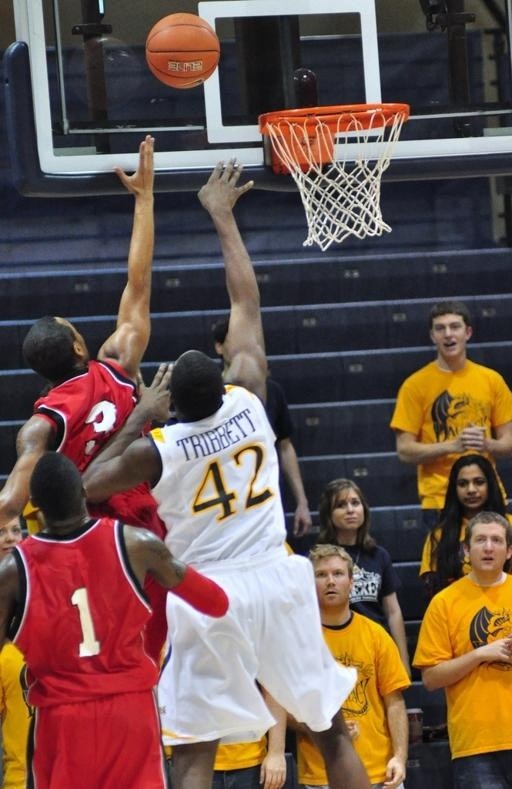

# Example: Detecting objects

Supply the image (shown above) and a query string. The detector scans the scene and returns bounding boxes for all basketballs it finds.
[146,13,220,89]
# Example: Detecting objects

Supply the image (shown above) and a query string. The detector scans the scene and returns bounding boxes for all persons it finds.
[164,478,413,788]
[411,510,512,789]
[1,133,168,660]
[1,452,229,788]
[416,453,512,598]
[84,156,373,788]
[389,300,512,529]
[0,500,44,788]
[211,316,312,537]
